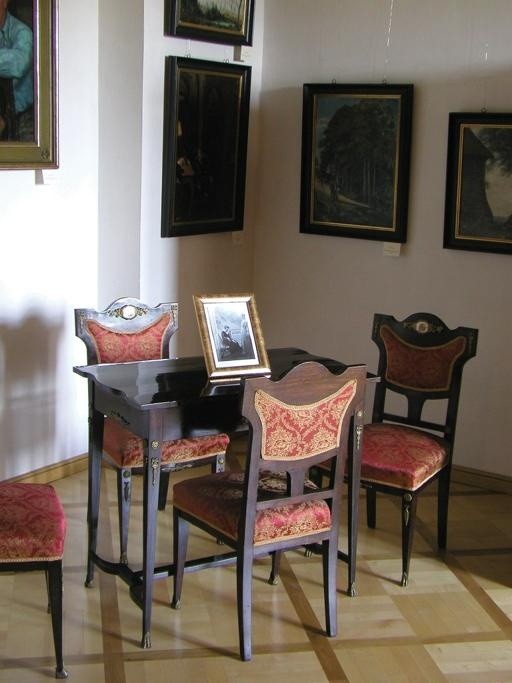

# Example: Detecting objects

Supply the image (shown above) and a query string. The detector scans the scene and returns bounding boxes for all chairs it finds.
[74,295,229,566]
[170,360,367,661]
[304,313,480,586]
[1,484,68,678]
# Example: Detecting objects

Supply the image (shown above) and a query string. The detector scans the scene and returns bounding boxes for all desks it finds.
[73,345,381,649]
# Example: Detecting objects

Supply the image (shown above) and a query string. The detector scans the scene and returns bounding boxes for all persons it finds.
[240,312,255,356]
[0,0,35,142]
[220,324,243,356]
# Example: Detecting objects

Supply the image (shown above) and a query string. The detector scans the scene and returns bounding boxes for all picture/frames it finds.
[163,1,255,48]
[160,55,252,239]
[299,81,414,245]
[190,293,273,384]
[442,112,512,254]
[0,0,60,169]
